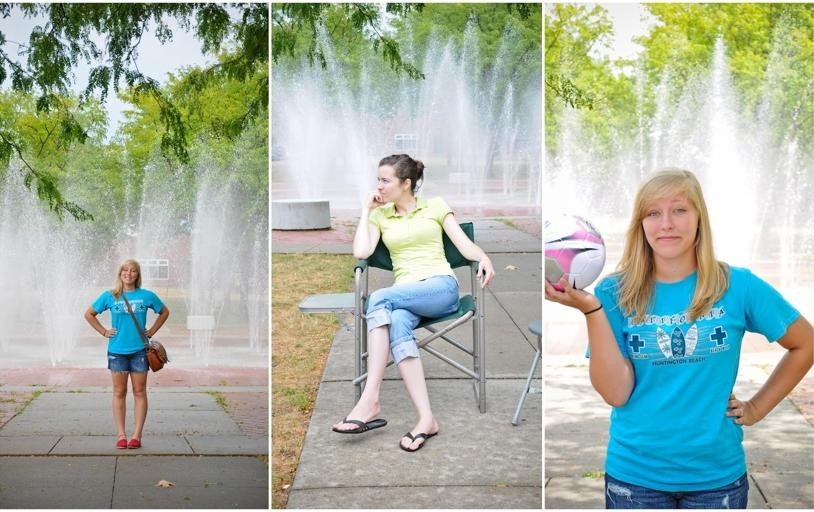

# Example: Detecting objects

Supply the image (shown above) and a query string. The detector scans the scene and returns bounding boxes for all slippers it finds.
[398,432,439,454]
[332,416,389,435]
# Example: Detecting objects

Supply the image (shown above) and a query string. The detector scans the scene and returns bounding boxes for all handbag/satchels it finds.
[144,340,171,374]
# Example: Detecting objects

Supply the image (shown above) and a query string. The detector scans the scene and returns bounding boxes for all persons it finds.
[545,165,814,509]
[331,152,497,452]
[83,259,170,449]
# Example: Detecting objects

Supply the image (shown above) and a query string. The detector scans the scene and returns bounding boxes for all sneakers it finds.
[116,433,143,450]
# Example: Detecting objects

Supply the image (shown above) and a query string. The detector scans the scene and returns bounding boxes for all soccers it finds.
[543,214,605,291]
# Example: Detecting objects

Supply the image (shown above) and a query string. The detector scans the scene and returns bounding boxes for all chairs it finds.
[349,220,489,416]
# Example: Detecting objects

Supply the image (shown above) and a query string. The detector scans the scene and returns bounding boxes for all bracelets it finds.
[584,303,603,316]
[103,330,106,337]
